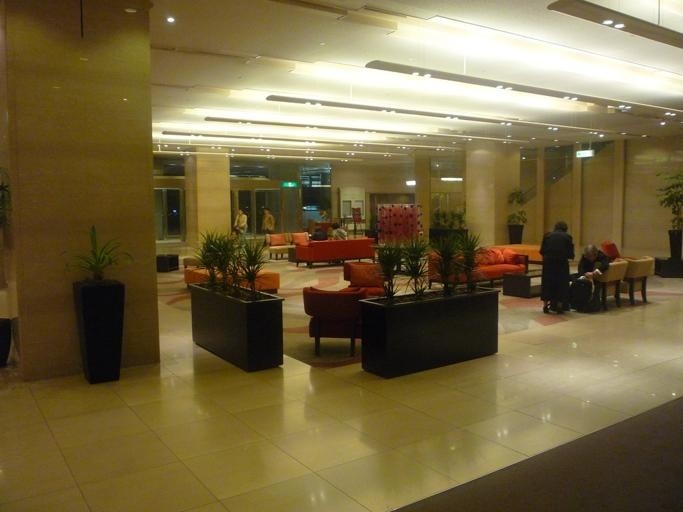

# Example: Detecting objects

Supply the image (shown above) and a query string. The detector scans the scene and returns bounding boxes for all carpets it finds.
[391,394,683,511]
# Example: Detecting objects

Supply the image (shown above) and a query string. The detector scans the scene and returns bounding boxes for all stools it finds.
[184,268,280,294]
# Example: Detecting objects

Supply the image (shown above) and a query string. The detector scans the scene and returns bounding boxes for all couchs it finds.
[266,232,309,260]
[428,248,528,289]
[295,238,375,268]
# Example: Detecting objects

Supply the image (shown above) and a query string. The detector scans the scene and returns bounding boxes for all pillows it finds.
[270,233,287,245]
[349,263,383,287]
[291,232,307,245]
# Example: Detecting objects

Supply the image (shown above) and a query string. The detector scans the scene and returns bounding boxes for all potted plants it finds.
[0,176,12,368]
[65,224,135,384]
[357,233,501,379]
[506,185,530,244]
[188,230,286,372]
[430,208,468,241]
[655,168,683,260]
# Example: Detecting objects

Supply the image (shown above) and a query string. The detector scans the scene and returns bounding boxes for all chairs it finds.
[621,255,655,305]
[597,258,628,310]
[303,261,385,357]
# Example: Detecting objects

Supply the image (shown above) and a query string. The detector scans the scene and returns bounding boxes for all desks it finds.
[503,270,543,298]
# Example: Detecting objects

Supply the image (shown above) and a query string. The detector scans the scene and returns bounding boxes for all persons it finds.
[306,222,328,266]
[539,221,574,315]
[569,273,600,313]
[261,208,275,247]
[233,209,248,235]
[331,223,347,265]
[579,245,612,278]
